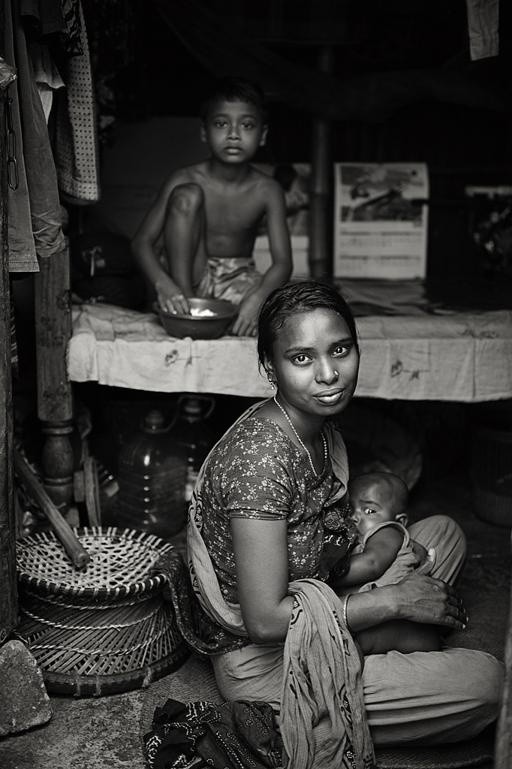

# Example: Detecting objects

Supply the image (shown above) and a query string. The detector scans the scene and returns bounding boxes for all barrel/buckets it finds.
[113,410,189,538]
[175,394,218,504]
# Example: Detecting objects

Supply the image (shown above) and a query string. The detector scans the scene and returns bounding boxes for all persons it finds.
[131,71,293,336]
[185,281,505,747]
[331,472,440,656]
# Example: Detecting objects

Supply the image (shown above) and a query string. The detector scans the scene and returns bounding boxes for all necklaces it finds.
[274,397,328,478]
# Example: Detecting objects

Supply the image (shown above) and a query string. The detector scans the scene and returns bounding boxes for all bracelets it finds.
[342,593,354,633]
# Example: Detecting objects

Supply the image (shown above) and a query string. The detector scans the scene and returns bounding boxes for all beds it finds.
[37,249,512,532]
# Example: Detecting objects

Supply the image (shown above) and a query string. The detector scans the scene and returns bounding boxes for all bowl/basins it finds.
[152,296,240,340]
